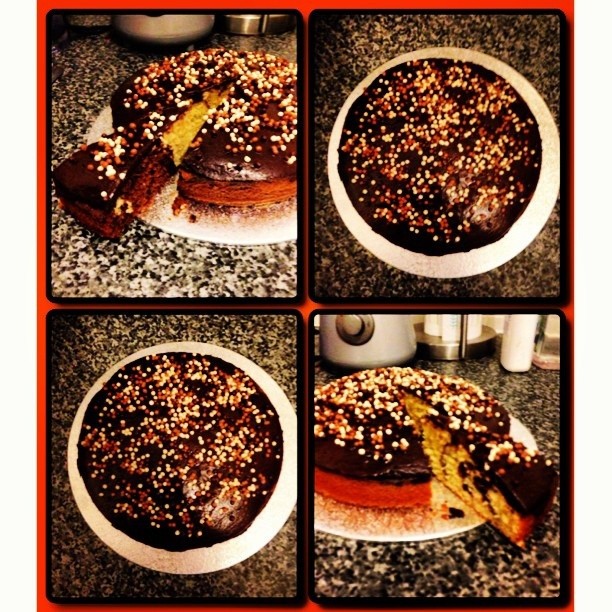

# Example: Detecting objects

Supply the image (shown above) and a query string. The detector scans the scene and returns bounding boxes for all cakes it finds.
[341,59,542,251]
[56,46,296,242]
[78,351,284,552]
[315,365,557,546]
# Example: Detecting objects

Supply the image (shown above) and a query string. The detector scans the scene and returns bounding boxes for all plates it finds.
[87,101,298,245]
[327,48,560,279]
[68,341,297,576]
[314,407,538,543]
